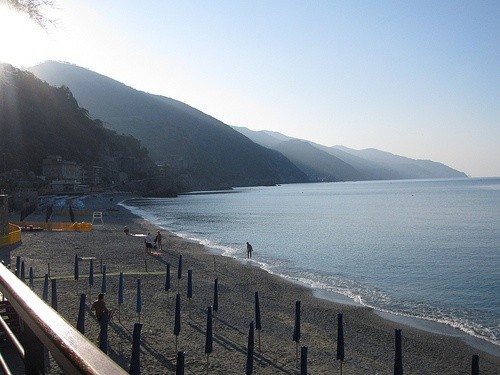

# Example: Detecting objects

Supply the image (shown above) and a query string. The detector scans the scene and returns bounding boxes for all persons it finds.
[145,232,153,254]
[246,242,253,258]
[156,231,163,250]
[91,293,111,340]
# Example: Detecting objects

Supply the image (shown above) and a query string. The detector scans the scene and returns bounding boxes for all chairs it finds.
[92,211,104,227]
